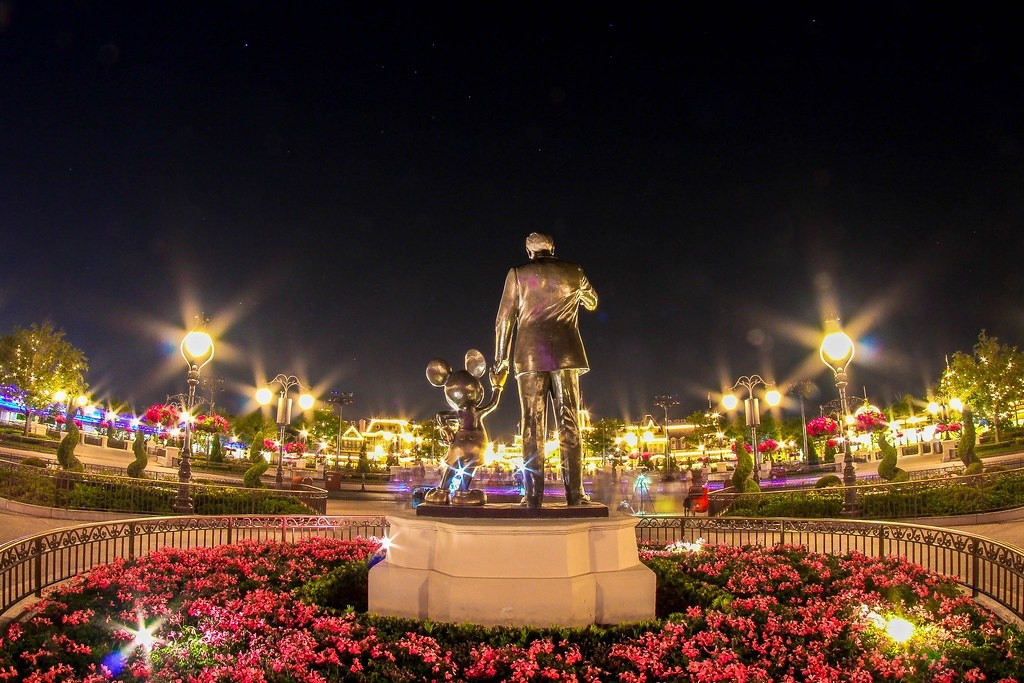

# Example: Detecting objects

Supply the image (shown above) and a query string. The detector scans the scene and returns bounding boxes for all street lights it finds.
[54,390,88,432]
[171,312,215,514]
[327,390,356,472]
[924,398,966,440]
[254,372,316,490]
[722,374,781,484]
[817,313,867,518]
[652,393,681,474]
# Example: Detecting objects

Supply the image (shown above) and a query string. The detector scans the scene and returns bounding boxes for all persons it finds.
[494,231,598,509]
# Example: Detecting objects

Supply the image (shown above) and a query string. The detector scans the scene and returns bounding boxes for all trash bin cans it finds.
[769,466,786,484]
[325,471,341,491]
